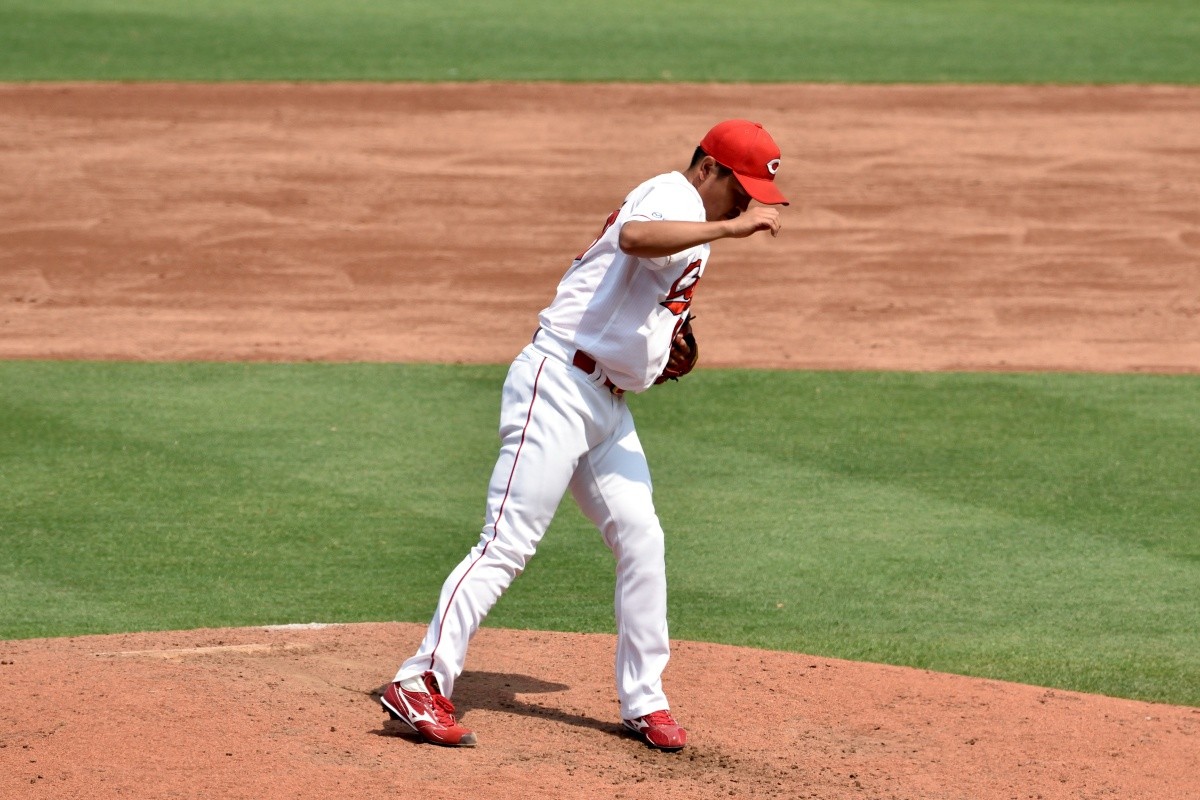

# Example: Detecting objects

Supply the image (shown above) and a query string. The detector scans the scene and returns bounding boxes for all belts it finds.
[531,327,627,397]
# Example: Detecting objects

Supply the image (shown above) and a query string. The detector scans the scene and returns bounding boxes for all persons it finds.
[381,118,790,749]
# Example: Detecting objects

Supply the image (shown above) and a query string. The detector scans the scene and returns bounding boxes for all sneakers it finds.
[623,707,688,749]
[381,672,478,749]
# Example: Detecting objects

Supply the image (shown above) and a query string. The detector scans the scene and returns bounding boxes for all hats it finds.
[699,119,790,207]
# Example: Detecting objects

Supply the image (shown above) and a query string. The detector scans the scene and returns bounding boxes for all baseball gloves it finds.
[654,311,700,385]
